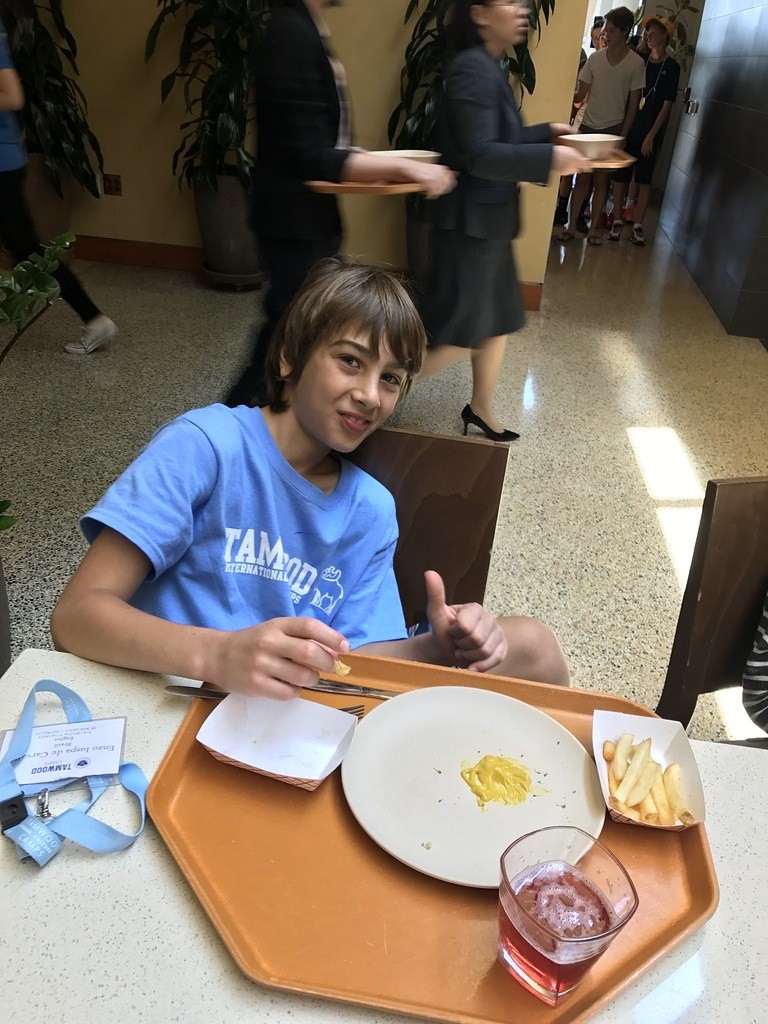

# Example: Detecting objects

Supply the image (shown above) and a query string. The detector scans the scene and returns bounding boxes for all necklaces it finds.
[639,52,668,110]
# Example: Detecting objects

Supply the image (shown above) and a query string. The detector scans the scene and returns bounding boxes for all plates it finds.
[341,685,607,888]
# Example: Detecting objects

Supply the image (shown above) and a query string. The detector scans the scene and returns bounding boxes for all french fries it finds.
[335,660,351,676]
[602,734,695,826]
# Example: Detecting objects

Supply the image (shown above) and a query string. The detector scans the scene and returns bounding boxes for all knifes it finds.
[281,676,404,699]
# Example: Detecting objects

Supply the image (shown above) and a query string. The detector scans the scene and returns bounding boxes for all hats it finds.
[643,14,674,38]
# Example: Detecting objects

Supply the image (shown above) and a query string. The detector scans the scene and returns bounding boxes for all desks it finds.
[0,648,768,1024]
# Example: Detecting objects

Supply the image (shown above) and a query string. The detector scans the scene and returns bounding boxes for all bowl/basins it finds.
[559,134,625,160]
[366,150,443,181]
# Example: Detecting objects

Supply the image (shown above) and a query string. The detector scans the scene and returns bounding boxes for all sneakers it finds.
[628,227,646,247]
[62,316,117,354]
[607,223,623,241]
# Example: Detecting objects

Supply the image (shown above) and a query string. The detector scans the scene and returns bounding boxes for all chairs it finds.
[340,425,511,671]
[655,476,768,749]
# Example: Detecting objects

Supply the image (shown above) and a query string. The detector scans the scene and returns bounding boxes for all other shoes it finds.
[558,231,575,242]
[587,233,602,245]
[554,204,644,234]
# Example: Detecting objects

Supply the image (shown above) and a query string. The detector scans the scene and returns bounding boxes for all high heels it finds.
[461,404,520,441]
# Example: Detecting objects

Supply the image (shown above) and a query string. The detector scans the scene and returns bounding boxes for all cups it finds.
[498,826,639,1008]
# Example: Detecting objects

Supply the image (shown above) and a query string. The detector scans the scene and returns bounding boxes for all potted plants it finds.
[388,0,556,304]
[145,0,289,291]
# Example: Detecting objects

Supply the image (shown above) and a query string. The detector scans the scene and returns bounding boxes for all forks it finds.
[164,685,365,718]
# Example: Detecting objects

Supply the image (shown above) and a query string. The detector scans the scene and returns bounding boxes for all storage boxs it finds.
[593,708,706,832]
[195,688,360,792]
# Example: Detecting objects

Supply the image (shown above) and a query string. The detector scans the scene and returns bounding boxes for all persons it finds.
[223,0,458,407]
[0,36,117,355]
[410,0,592,443]
[50,260,571,698]
[553,5,682,246]
